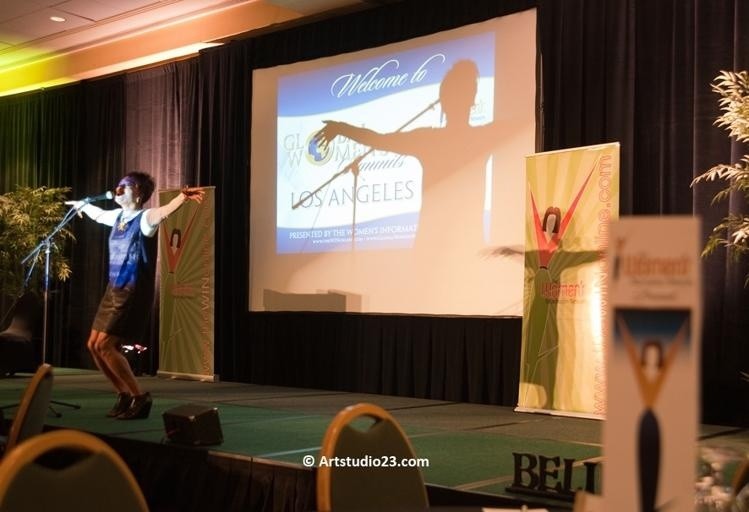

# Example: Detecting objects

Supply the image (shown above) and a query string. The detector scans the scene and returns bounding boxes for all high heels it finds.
[108,392,131,417]
[118,392,153,419]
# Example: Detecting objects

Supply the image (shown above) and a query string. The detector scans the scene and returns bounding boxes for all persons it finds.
[62,171,207,422]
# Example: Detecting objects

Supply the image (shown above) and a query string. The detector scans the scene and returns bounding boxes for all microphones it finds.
[81,190,115,203]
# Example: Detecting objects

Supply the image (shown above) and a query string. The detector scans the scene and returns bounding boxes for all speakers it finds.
[161,404,224,447]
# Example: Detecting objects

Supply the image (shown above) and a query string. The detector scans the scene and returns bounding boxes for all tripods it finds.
[1,203,82,418]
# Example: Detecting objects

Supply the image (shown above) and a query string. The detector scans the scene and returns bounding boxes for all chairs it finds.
[0,363,150,512]
[315,403,430,512]
